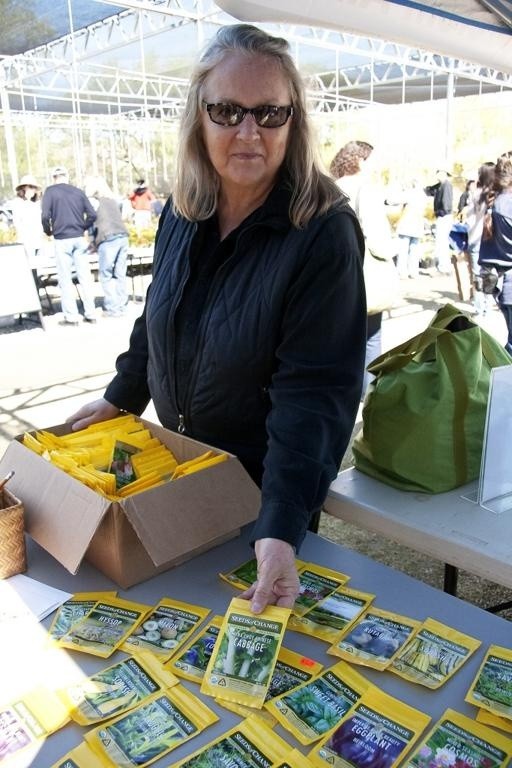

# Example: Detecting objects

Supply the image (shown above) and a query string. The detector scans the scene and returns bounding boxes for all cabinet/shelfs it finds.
[0,236,48,336]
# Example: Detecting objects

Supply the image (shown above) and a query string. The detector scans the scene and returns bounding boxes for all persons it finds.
[40,167,97,325]
[68,22,369,616]
[331,140,398,339]
[0,176,43,299]
[423,155,512,356]
[128,176,153,234]
[83,186,129,312]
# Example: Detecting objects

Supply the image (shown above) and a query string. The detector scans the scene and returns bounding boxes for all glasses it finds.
[202,99,294,129]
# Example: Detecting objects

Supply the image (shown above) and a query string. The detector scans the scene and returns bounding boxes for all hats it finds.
[52,165,67,176]
[15,175,43,193]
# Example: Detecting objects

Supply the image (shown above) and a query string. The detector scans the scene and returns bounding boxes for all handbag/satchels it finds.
[351,308,512,494]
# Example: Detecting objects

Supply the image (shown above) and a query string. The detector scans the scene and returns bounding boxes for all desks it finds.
[0,516,510,766]
[324,461,511,617]
[28,243,156,319]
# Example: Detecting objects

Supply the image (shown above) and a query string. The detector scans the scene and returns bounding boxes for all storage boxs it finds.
[1,403,262,594]
